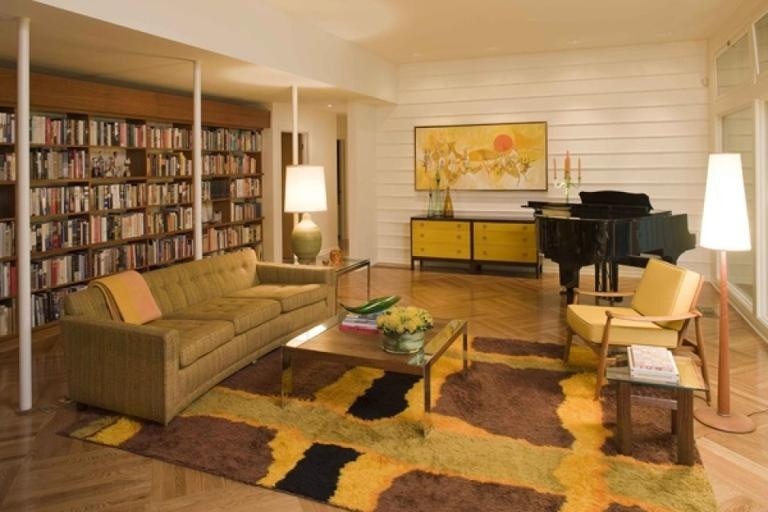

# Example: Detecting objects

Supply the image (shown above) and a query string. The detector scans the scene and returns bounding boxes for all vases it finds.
[386,334,425,355]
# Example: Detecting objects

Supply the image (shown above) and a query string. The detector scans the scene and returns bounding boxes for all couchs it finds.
[60,250,337,427]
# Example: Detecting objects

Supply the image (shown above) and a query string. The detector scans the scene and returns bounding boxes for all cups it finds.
[330,249,343,265]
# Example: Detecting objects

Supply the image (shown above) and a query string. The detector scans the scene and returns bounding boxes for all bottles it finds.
[444,187,453,218]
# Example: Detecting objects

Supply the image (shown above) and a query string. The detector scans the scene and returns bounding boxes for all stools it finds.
[605,352,710,466]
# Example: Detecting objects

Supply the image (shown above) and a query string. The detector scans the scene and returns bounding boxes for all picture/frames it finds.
[413,121,548,191]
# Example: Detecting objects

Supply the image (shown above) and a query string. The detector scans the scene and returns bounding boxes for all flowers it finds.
[377,307,434,335]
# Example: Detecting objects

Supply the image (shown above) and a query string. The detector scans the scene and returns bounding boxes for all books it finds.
[627,344,679,384]
[201,126,262,257]
[0,113,19,339]
[28,115,193,327]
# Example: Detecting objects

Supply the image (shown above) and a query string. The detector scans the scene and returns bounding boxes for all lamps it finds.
[284,166,328,265]
[694,154,754,434]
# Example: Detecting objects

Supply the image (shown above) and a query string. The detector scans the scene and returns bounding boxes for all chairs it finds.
[563,258,713,407]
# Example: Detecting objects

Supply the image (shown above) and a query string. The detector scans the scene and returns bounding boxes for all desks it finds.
[410,212,542,279]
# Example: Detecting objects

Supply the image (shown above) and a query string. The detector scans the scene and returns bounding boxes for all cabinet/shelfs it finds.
[0,68,271,348]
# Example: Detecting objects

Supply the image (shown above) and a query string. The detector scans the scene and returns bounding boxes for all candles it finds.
[578,159,580,178]
[553,159,556,178]
[567,151,569,173]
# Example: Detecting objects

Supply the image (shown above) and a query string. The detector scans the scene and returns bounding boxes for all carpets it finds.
[56,336,720,512]
